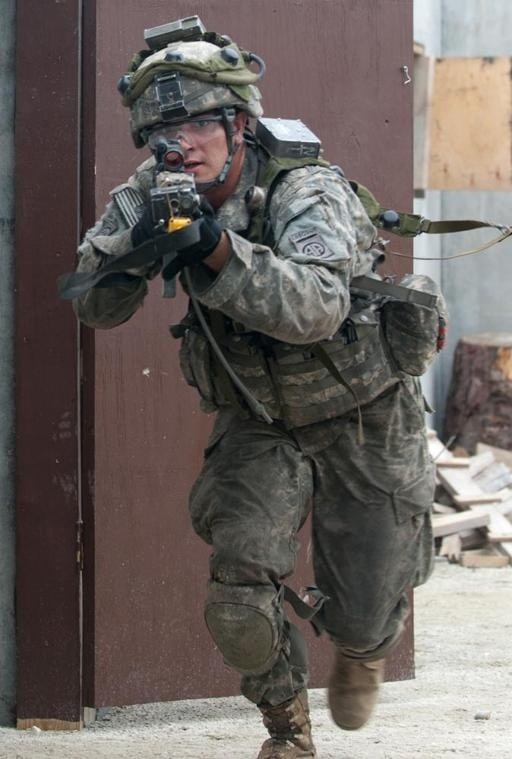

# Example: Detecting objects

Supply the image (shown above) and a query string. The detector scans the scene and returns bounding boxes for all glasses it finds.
[138,114,221,152]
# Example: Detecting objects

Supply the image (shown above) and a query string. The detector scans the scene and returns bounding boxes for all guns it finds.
[149,134,201,297]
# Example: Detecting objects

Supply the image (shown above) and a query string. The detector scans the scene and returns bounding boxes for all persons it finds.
[65,8,449,758]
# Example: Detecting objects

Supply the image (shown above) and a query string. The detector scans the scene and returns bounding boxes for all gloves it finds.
[131,198,219,279]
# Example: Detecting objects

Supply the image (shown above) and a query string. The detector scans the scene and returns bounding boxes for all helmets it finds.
[127,37,264,150]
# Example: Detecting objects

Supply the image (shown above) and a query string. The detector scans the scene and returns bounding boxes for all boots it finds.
[327,652,386,731]
[257,687,318,759]
